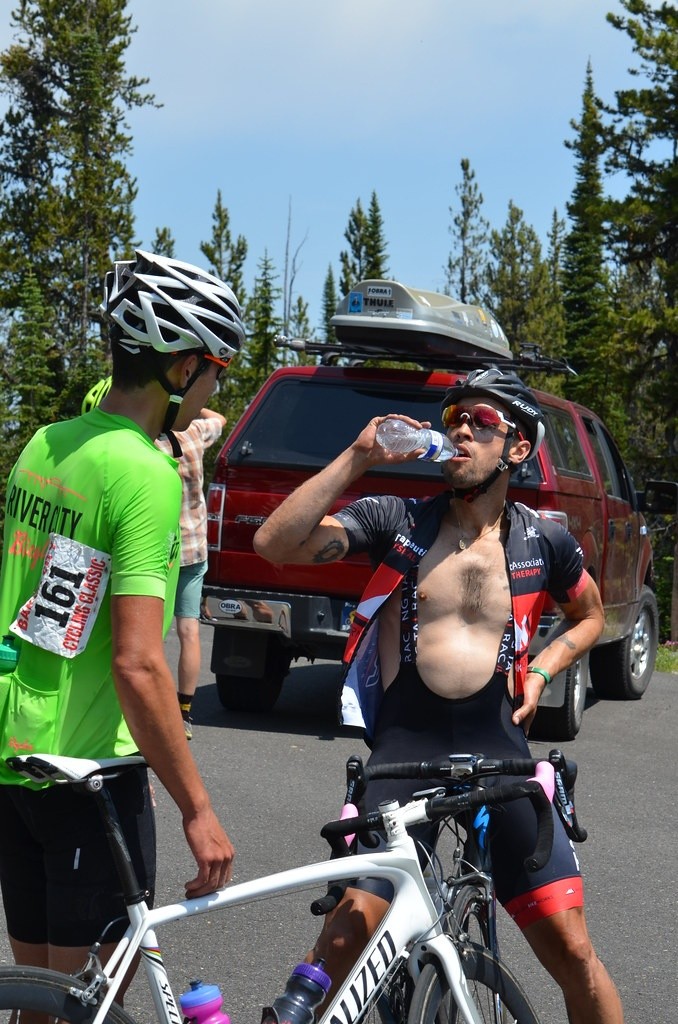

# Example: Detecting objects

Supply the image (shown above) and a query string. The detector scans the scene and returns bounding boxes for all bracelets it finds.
[527,667,552,684]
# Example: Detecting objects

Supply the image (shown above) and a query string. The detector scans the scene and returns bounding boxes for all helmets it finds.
[81,374,112,416]
[94,249,246,366]
[439,367,545,463]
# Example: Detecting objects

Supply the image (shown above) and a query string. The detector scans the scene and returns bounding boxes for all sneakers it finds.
[179,709,193,738]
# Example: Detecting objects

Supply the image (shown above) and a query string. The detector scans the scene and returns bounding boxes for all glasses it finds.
[443,402,526,443]
[217,367,226,379]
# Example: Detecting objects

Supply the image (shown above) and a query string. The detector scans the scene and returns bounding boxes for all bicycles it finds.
[0,780,554,1024]
[341,749,588,1024]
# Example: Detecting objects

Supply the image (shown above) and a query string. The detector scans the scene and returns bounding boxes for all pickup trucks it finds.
[199,336,659,743]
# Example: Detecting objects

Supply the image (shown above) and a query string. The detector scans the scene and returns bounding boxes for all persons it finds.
[0,249,245,1024]
[253,364,622,1024]
[82,374,227,740]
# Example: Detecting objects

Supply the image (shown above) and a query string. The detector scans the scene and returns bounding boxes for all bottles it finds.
[272,957,332,1024]
[0,634,18,722]
[375,419,459,463]
[179,980,232,1024]
